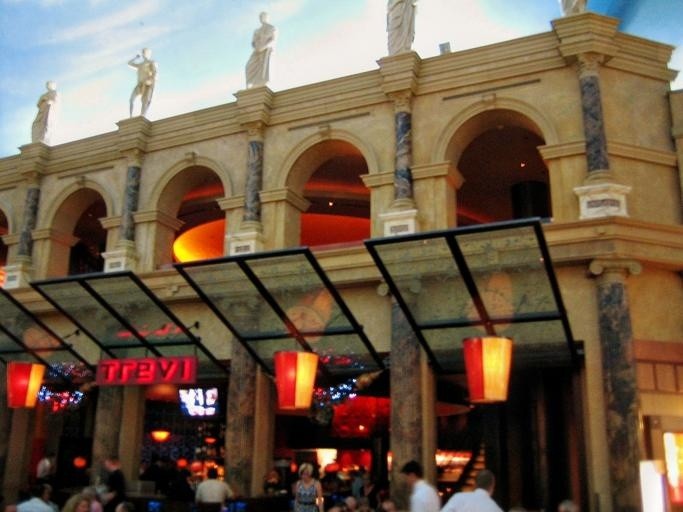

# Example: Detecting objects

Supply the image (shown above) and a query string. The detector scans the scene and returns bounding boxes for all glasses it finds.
[6,354,46,410]
[273,338,319,411]
[462,324,513,404]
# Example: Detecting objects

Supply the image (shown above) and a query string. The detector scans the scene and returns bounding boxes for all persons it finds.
[125,48,156,119]
[30,81,59,146]
[242,11,276,90]
[384,1,419,55]
